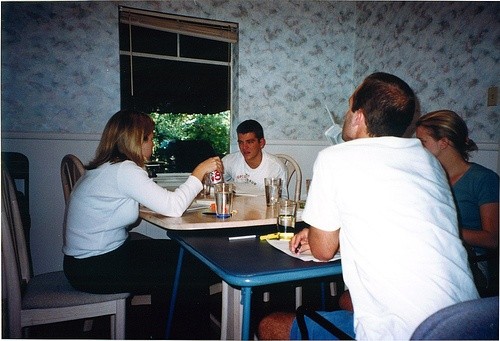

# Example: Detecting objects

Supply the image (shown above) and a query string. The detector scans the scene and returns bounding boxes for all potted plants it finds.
[144,139,161,178]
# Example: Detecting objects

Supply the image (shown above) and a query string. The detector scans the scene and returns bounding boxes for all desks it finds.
[163,230,342,341]
[140,193,305,230]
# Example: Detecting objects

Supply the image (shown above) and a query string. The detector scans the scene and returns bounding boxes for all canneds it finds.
[209,170,224,184]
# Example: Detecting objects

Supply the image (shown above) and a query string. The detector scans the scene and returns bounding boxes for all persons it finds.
[417,110,500,298]
[257,71,479,341]
[220,120,289,200]
[64,110,225,340]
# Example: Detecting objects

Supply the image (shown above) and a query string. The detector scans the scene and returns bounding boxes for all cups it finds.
[214,183,233,219]
[264,178,283,207]
[275,201,297,241]
[305,180,312,196]
[201,173,212,195]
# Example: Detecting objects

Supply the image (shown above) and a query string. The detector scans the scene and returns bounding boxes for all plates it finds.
[156,173,191,187]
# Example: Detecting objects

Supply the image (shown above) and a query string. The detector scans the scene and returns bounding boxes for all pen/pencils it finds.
[228,234,258,240]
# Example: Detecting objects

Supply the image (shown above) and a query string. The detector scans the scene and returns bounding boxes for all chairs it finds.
[295,296,499,340]
[274,154,300,201]
[1,160,130,339]
[60,154,84,201]
